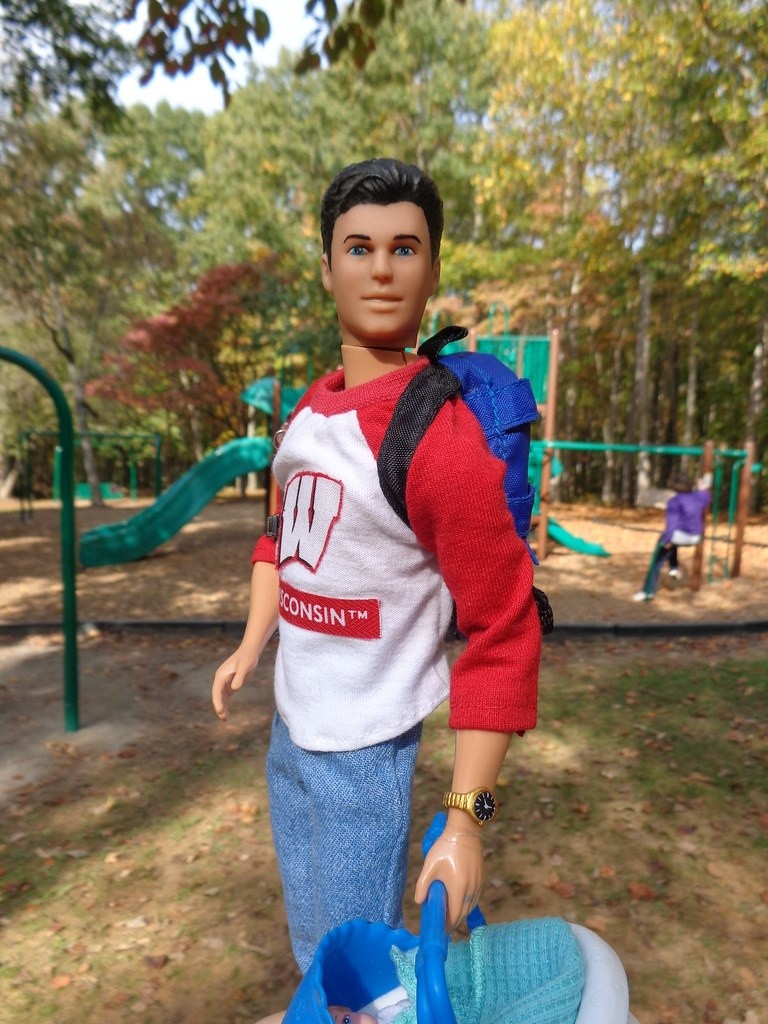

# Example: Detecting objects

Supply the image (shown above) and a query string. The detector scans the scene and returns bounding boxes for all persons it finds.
[630,470,711,602]
[326,918,584,1024]
[212,151,539,983]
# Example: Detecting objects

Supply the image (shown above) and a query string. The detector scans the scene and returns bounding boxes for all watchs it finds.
[442,786,499,828]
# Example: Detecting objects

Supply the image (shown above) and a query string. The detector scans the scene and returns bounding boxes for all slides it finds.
[548,515,612,558]
[78,437,276,568]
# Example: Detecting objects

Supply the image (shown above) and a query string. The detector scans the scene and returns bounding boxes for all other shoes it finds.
[633,590,654,603]
[668,570,678,575]
